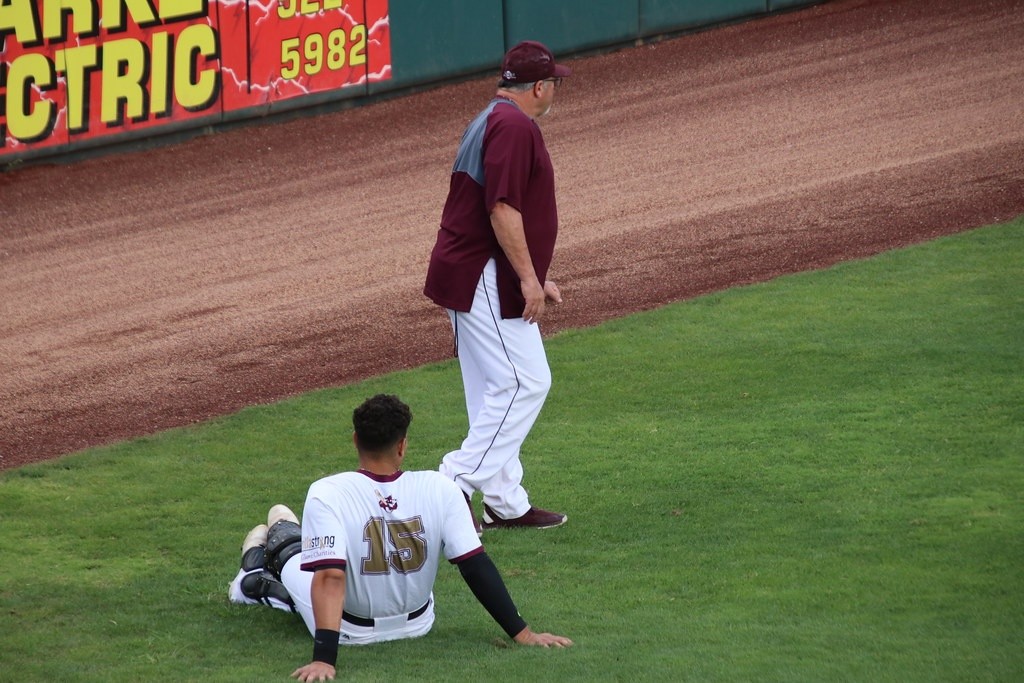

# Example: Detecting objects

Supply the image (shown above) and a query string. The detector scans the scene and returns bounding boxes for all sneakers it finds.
[228,524,270,605]
[480,501,567,530]
[268,504,300,528]
[463,491,484,538]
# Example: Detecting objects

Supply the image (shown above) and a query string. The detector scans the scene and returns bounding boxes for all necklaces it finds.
[358,466,400,476]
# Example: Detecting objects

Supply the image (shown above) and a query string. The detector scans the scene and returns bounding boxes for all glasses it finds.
[533,77,563,87]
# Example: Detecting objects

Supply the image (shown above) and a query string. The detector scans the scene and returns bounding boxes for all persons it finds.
[228,395,574,683]
[420,40,567,537]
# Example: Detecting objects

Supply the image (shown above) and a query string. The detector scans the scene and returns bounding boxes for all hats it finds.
[501,41,572,84]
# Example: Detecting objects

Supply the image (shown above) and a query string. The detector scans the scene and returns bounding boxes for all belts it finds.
[342,599,430,627]
